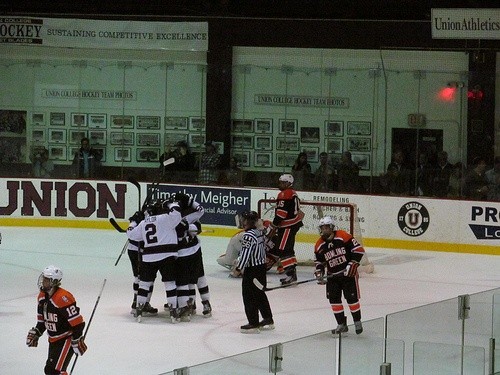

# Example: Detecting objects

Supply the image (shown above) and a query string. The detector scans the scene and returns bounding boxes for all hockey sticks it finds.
[253,269,346,291]
[109,158,176,265]
[69,279,106,375]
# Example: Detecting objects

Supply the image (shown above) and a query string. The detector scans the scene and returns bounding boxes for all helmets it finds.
[146,201,162,217]
[242,210,258,228]
[42,266,63,288]
[279,174,294,183]
[318,216,336,227]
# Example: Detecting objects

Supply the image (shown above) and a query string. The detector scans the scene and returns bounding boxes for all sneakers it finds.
[354,321,363,334]
[137,303,144,323]
[200,300,213,318]
[163,303,169,311]
[240,325,260,334]
[331,324,351,337]
[280,270,299,287]
[169,304,192,323]
[259,319,275,329]
[130,301,159,316]
[189,301,197,314]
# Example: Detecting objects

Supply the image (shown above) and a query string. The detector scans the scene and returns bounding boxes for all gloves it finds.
[265,222,277,238]
[344,262,361,278]
[175,193,193,208]
[313,270,327,285]
[176,221,189,238]
[25,326,43,348]
[70,336,87,356]
[129,211,144,225]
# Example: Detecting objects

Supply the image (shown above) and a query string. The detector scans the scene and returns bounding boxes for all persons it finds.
[314,217,365,335]
[71,138,101,180]
[129,199,183,326]
[156,139,242,188]
[273,174,304,285]
[25,265,87,375]
[229,210,274,333]
[126,203,158,317]
[33,148,55,179]
[385,147,500,204]
[163,192,211,322]
[291,149,364,195]
